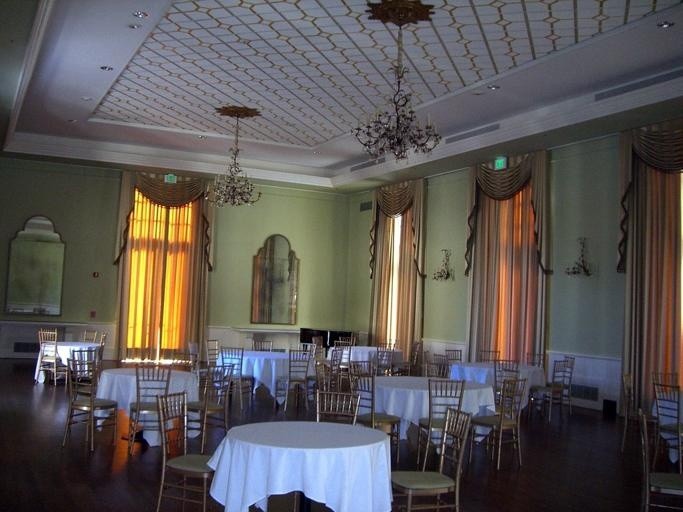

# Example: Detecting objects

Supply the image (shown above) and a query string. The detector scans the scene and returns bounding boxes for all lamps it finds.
[351,1,442,161]
[203,105,262,208]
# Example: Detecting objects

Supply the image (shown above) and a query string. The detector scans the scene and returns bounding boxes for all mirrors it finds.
[3,215,66,317]
[250,233,301,327]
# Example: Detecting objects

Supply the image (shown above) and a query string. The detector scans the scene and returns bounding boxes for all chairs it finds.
[528,359,568,424]
[432,354,448,378]
[186,364,234,455]
[546,355,576,415]
[318,350,343,391]
[83,330,97,343]
[216,346,254,410]
[339,336,356,346]
[272,348,311,413]
[392,406,473,512]
[62,358,118,451]
[334,340,351,372]
[315,389,361,426]
[34,331,68,387]
[374,342,394,376]
[170,352,198,373]
[403,342,421,376]
[312,335,323,358]
[129,363,172,456]
[155,390,214,512]
[416,378,466,472]
[98,332,107,360]
[297,343,316,357]
[349,360,371,376]
[445,349,462,364]
[469,378,527,470]
[493,359,520,405]
[72,349,97,401]
[652,371,679,397]
[40,328,58,341]
[252,339,273,352]
[479,350,500,361]
[203,339,220,396]
[348,373,401,465]
[652,381,683,473]
[188,342,219,379]
[638,408,683,512]
[620,373,657,453]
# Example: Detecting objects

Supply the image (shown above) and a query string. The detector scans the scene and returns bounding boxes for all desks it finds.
[215,351,316,406]
[95,367,221,448]
[450,361,545,411]
[205,420,393,512]
[34,341,101,385]
[357,376,495,456]
[652,391,683,464]
[326,346,403,375]
